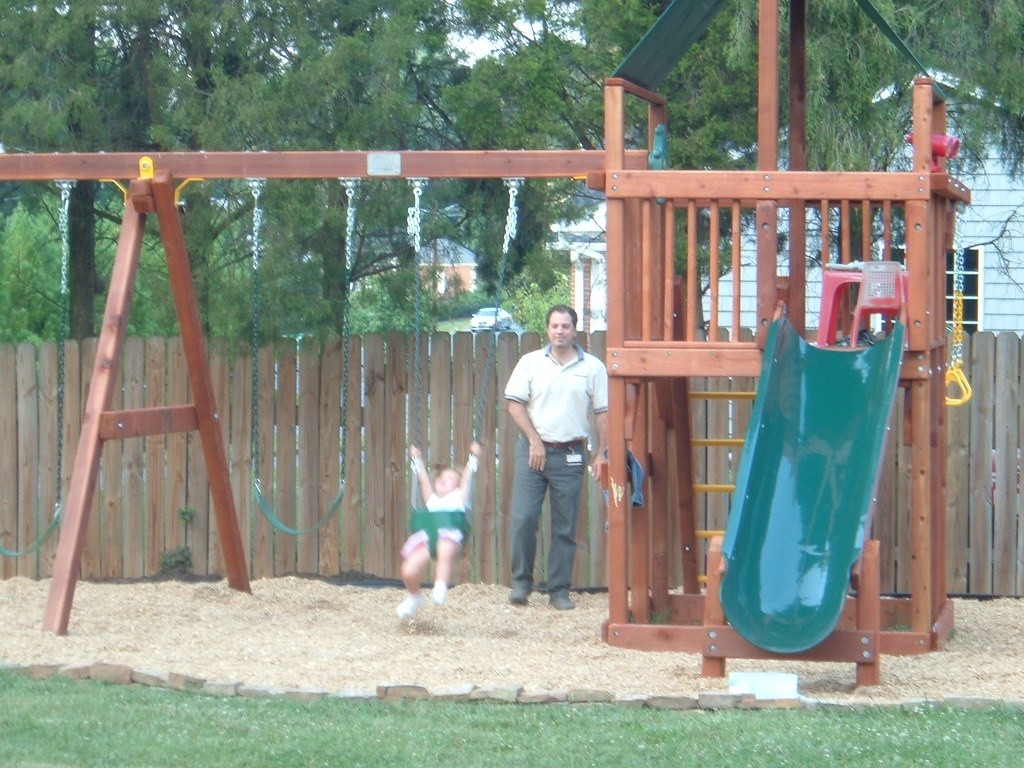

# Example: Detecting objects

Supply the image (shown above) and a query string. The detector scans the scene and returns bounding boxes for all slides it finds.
[716,314,903,656]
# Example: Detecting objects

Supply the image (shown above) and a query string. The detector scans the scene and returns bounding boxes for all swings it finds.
[249,182,353,535]
[0,187,73,557]
[410,184,518,560]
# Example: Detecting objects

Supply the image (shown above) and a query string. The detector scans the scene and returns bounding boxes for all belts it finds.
[542,440,582,447]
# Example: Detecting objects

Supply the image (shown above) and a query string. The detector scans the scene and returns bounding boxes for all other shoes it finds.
[396,594,418,620]
[432,581,447,604]
[510,587,528,605]
[549,593,575,610]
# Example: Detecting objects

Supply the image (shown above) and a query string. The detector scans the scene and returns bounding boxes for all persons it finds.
[505,304,608,610]
[396,440,481,618]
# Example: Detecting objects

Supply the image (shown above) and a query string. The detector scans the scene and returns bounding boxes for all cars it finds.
[469,307,512,333]
